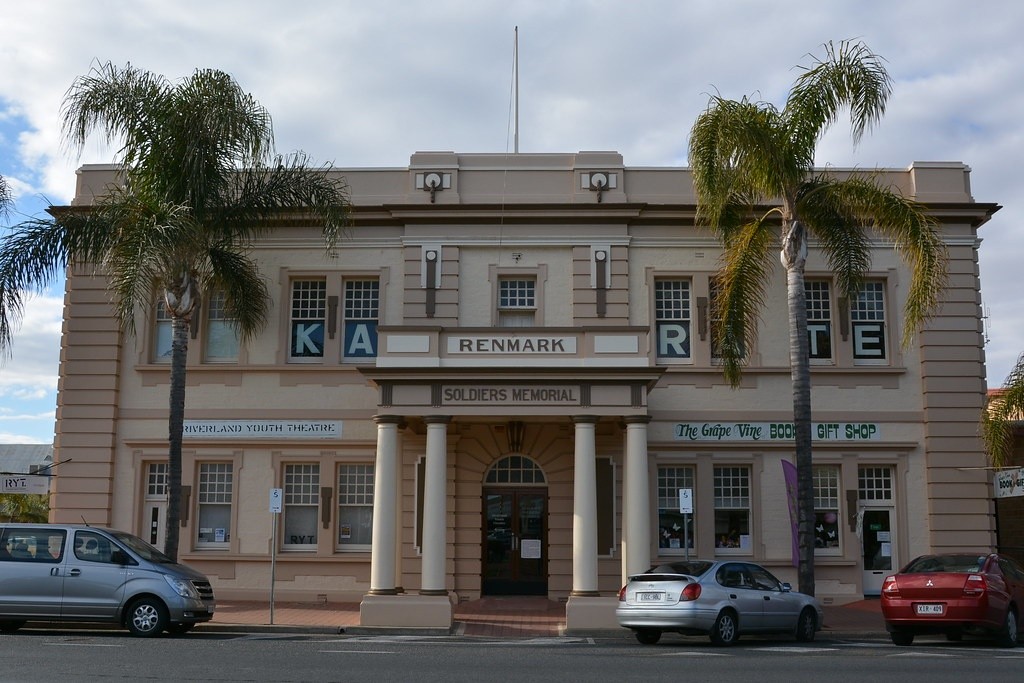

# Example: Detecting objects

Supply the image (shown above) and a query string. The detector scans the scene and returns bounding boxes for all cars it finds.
[614,559,823,647]
[880,550,1024,649]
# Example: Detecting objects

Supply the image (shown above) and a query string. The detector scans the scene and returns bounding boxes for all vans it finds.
[0,522,216,638]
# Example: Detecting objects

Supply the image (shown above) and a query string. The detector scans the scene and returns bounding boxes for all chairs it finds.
[11,543,32,558]
[85,540,99,560]
[74,538,85,559]
